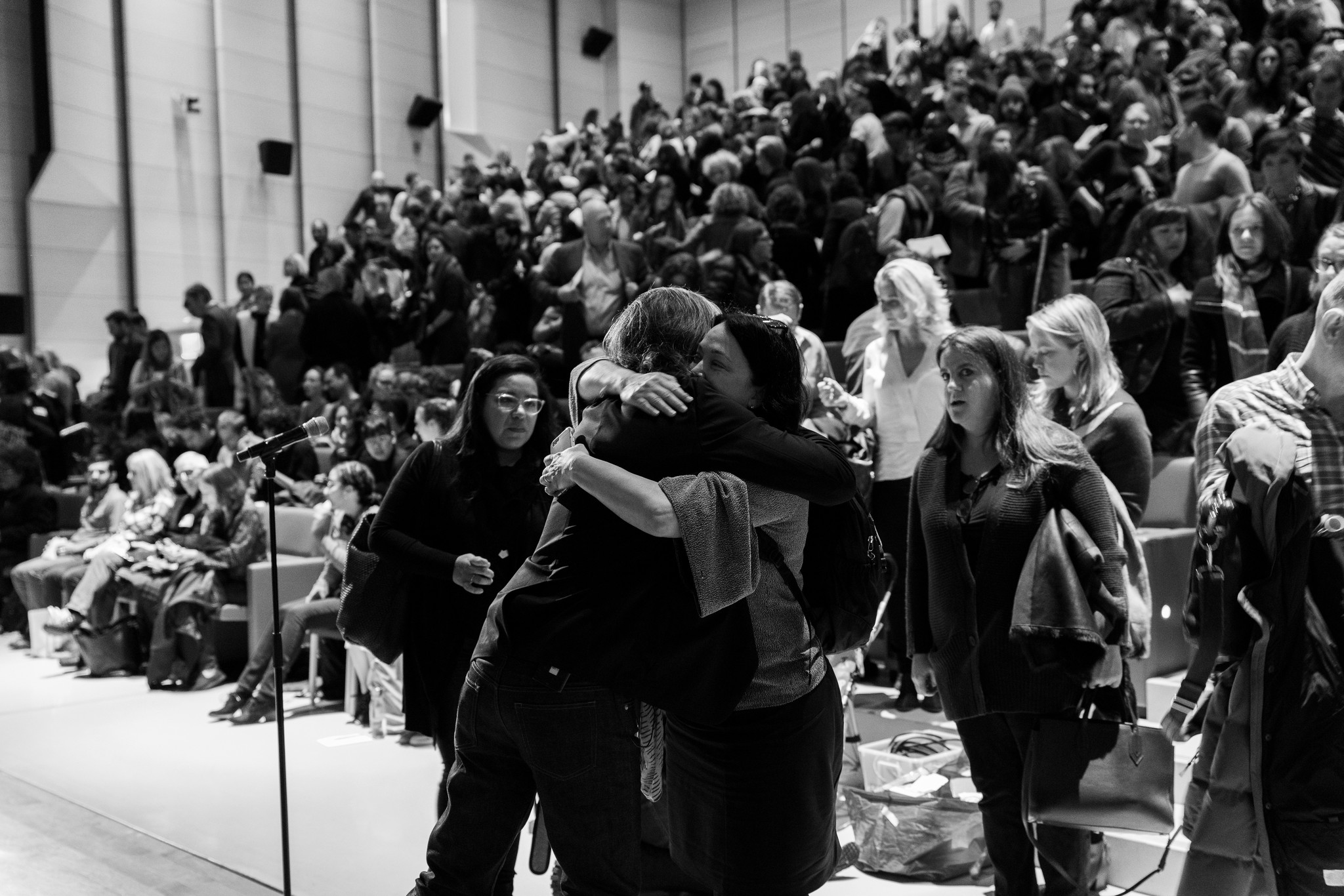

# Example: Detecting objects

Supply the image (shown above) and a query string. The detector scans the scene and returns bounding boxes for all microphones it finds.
[237,417,332,463]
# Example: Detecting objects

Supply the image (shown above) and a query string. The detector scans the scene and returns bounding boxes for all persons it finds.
[401,285,855,896]
[350,356,561,896]
[1167,266,1344,896]
[1018,291,1153,893]
[0,0,1344,734]
[902,323,1132,896]
[538,307,847,896]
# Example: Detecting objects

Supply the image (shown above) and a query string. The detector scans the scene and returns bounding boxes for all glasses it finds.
[173,469,196,480]
[484,390,546,417]
[1307,255,1344,275]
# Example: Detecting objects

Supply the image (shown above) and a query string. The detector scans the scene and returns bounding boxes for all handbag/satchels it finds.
[1027,664,1176,836]
[120,401,152,434]
[335,508,415,663]
[75,616,146,675]
[1179,464,1264,661]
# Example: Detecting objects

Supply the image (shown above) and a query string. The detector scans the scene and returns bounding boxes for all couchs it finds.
[1133,455,1200,683]
[227,502,341,685]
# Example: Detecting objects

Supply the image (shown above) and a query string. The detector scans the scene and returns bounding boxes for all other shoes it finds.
[189,664,227,693]
[44,613,88,635]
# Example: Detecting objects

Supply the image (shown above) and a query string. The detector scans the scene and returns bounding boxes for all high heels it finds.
[209,688,250,719]
[230,696,276,724]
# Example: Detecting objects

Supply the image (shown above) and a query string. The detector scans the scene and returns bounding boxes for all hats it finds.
[996,72,1028,105]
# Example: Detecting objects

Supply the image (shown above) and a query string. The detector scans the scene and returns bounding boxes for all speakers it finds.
[582,25,613,59]
[260,140,292,175]
[406,93,445,130]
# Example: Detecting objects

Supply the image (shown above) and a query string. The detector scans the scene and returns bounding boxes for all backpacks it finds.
[834,187,916,301]
[754,485,901,655]
